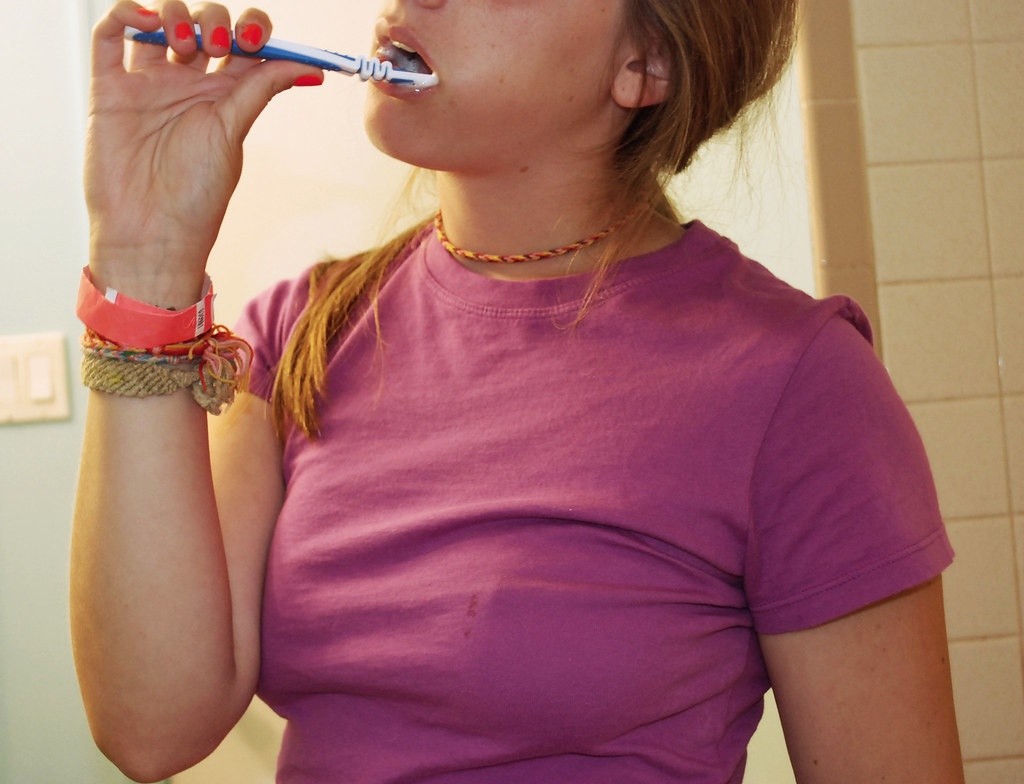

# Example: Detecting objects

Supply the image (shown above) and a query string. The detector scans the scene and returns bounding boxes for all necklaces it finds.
[434,208,639,263]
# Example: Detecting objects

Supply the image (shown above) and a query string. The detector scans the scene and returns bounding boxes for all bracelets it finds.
[76,265,254,416]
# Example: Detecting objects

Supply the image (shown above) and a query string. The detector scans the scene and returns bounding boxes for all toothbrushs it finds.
[121,20,441,92]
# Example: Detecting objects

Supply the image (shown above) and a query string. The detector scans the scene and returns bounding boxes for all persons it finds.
[72,0,965,784]
